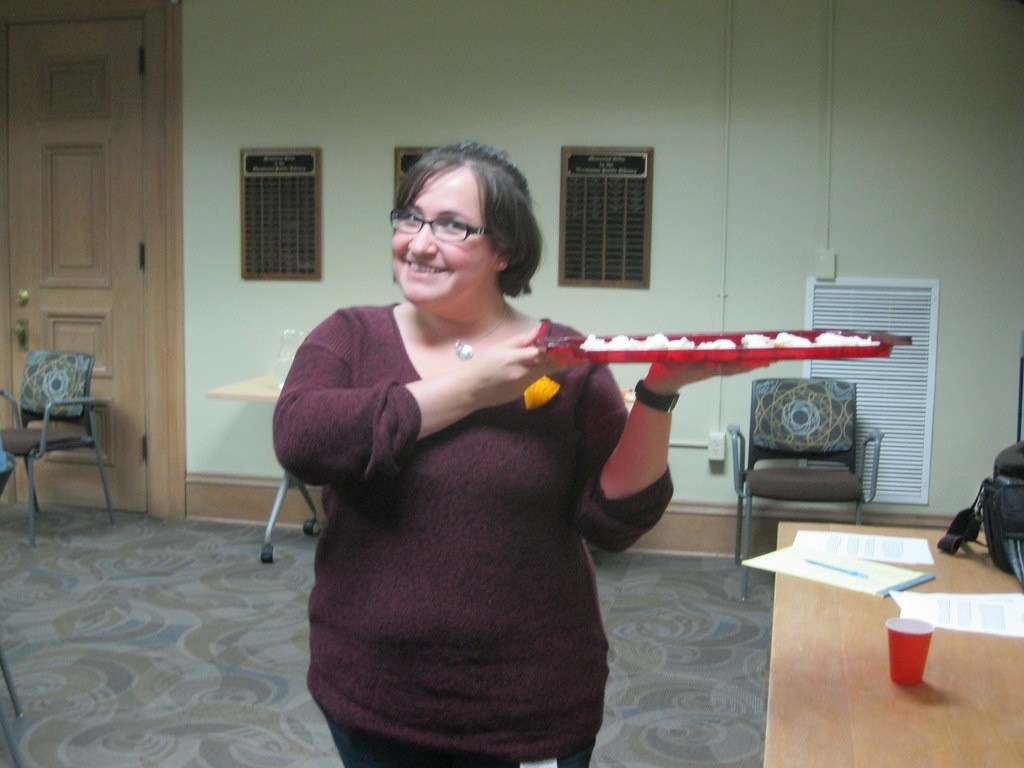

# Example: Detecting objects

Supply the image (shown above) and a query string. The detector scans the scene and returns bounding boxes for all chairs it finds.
[0,349,116,546]
[730,377,884,603]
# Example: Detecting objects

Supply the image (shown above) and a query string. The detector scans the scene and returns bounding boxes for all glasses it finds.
[389,207,487,241]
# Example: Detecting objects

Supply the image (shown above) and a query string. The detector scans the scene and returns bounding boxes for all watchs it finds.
[633,380,682,413]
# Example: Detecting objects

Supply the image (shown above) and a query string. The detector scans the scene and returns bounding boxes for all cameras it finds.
[983,475,1024,575]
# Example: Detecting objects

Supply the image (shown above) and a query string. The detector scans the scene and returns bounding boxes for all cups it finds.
[278,328,303,388]
[885,618,935,686]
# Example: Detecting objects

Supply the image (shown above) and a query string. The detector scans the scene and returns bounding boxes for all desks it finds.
[762,522,1024,768]
[207,376,319,565]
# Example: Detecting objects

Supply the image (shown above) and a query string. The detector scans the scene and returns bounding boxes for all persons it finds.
[270,141,773,768]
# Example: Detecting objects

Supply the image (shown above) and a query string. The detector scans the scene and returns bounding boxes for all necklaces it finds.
[410,294,509,359]
